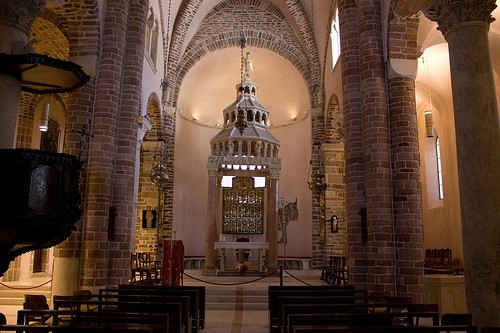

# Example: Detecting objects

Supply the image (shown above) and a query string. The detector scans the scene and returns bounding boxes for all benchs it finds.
[267,285,478,333]
[0,284,206,333]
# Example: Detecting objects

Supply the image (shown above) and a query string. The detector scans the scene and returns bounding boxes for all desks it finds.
[425,277,467,313]
[214,241,270,271]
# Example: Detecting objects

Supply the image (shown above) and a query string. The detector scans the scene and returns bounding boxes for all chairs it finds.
[369,294,500,333]
[320,256,348,285]
[0,289,92,333]
[130,252,162,283]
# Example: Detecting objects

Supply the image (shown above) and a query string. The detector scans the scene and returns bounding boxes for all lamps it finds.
[39,102,50,132]
[421,56,434,137]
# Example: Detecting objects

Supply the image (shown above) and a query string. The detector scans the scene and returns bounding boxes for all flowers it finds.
[235,263,248,271]
